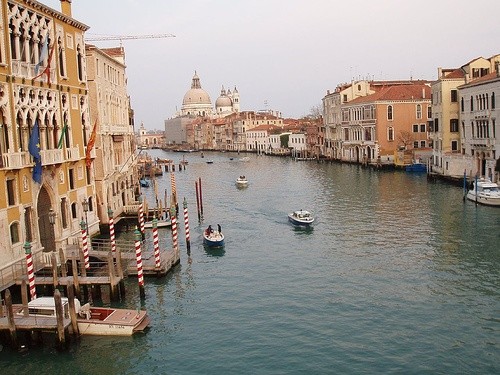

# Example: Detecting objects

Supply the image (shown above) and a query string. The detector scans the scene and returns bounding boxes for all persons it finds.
[78,301,92,319]
[207,225,214,235]
[217,224,222,237]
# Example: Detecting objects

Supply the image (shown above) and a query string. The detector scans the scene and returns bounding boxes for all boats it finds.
[180,154,188,163]
[139,178,149,187]
[288,210,315,226]
[202,227,225,248]
[236,176,248,184]
[230,157,233,160]
[206,161,213,164]
[239,157,250,162]
[466,178,500,205]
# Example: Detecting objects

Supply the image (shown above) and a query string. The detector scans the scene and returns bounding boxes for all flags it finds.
[28,122,42,184]
[34,38,47,75]
[86,121,97,170]
